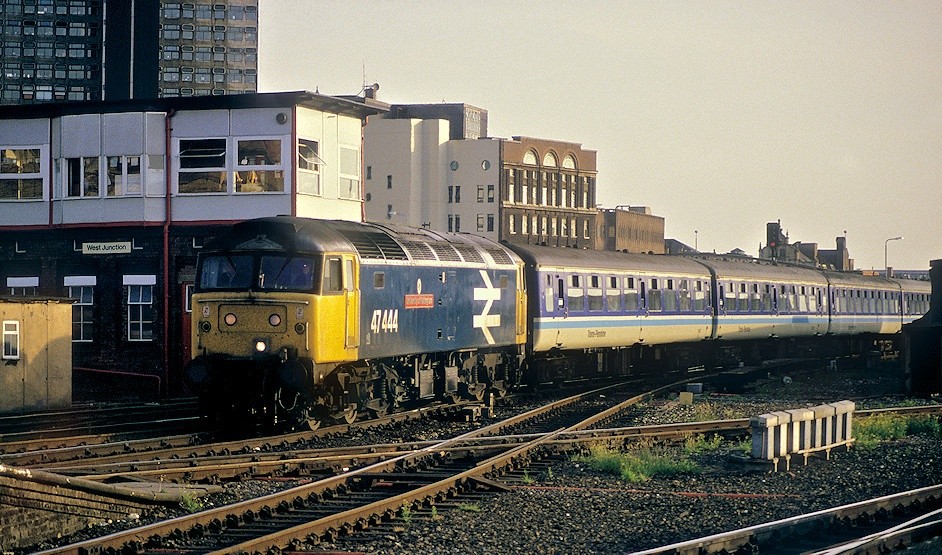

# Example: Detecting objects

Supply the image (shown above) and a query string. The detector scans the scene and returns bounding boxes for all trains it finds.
[190,215,932,433]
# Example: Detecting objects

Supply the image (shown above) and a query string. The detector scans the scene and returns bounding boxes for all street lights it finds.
[694,230,698,252]
[884,236,905,278]
